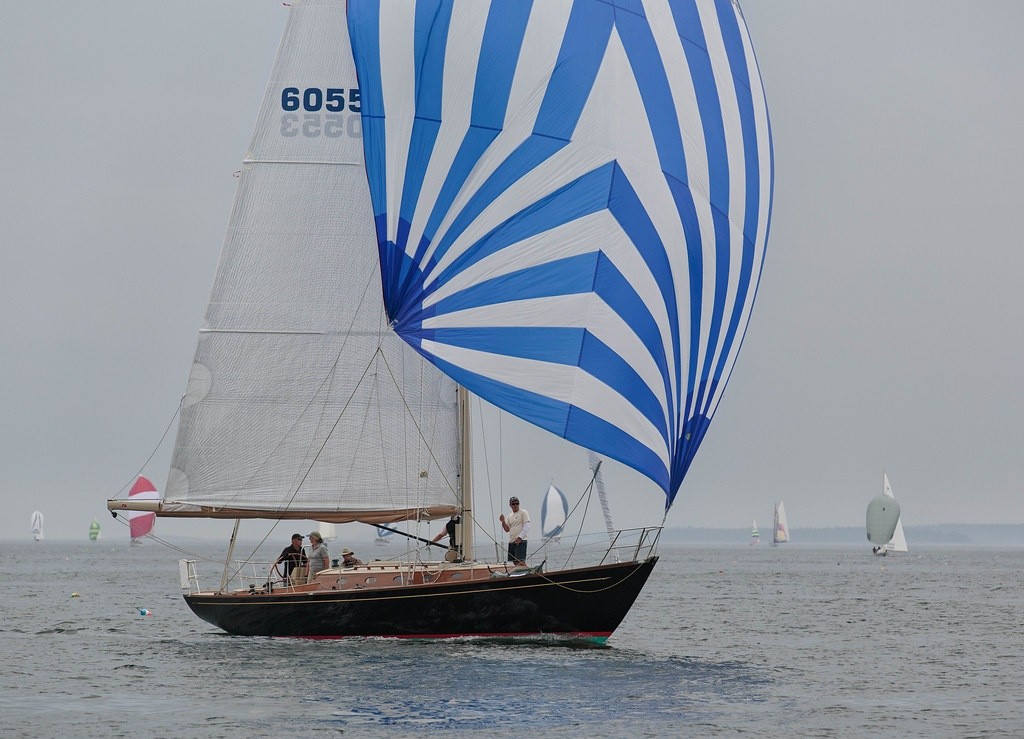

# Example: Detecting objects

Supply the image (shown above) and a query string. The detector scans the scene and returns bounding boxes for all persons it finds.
[431,514,462,563]
[340,548,363,568]
[873,546,881,554]
[306,531,330,584]
[499,496,530,565]
[277,534,308,587]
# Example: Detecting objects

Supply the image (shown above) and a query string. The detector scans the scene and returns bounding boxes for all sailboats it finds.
[540,483,568,543]
[127,475,159,546]
[768,499,791,548]
[748,519,760,546]
[31,510,43,542]
[375,523,396,541]
[866,469,908,555]
[89,516,100,543]
[318,521,337,542]
[106,0,775,645]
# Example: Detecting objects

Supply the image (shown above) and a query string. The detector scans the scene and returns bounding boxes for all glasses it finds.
[511,503,519,505]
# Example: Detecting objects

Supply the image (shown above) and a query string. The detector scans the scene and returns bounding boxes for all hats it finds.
[510,497,519,503]
[306,531,321,540]
[340,547,354,555]
[292,534,305,539]
